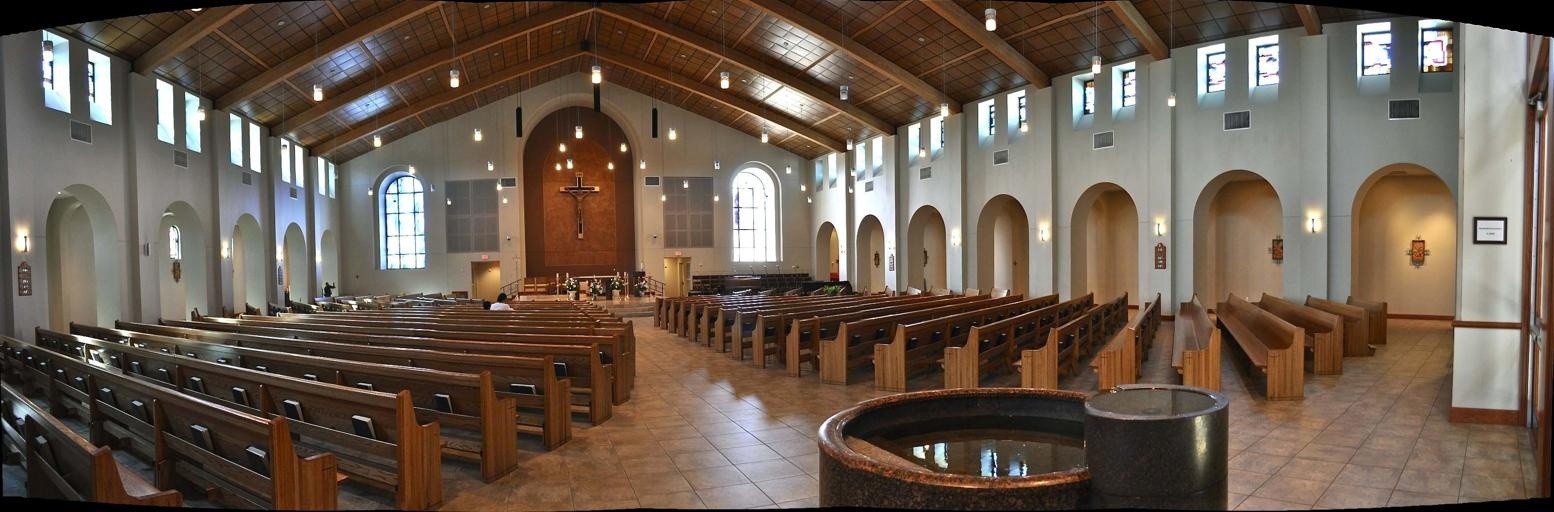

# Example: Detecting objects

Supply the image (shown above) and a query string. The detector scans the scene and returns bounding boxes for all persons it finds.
[490,293,513,311]
[482,299,491,310]
[324,282,335,297]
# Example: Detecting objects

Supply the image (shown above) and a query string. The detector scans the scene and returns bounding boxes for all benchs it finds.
[653,286,1387,400]
[0,292,636,511]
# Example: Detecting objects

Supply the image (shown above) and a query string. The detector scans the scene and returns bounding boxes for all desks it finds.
[572,275,616,300]
